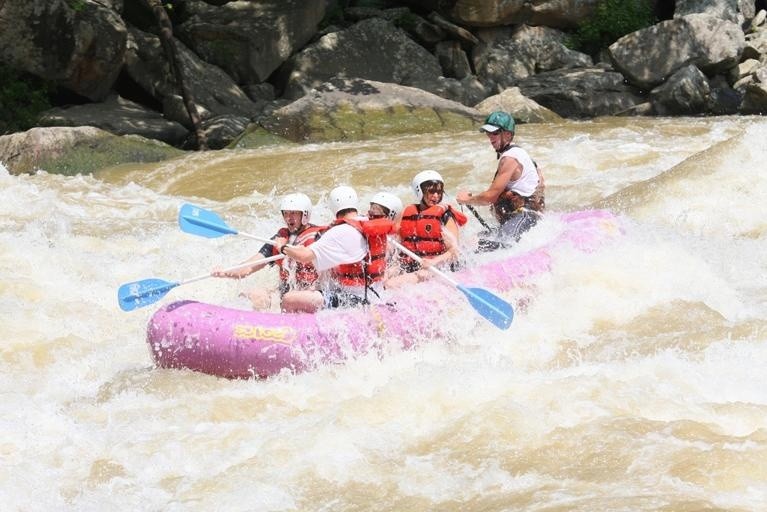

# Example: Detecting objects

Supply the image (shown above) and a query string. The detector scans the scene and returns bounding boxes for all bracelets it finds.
[281,245,288,255]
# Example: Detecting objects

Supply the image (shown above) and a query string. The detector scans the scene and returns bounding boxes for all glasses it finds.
[426,189,443,194]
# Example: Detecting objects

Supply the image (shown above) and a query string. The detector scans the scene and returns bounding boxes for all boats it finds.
[148,209,620,383]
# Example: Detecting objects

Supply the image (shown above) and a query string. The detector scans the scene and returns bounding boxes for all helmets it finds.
[329,186,358,218]
[485,112,515,134]
[370,192,403,218]
[412,170,444,201]
[281,193,312,226]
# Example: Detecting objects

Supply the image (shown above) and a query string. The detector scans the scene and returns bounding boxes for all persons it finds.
[456,111,546,247]
[368,191,404,224]
[211,192,330,310]
[273,185,387,313]
[382,169,467,290]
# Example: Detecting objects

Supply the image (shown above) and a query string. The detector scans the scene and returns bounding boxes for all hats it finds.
[479,123,501,133]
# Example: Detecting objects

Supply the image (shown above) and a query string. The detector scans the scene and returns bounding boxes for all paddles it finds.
[386,234,513,330]
[179,203,295,249]
[118,253,287,311]
